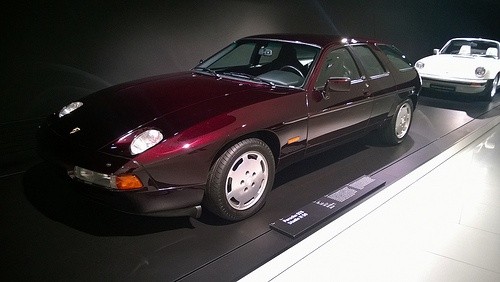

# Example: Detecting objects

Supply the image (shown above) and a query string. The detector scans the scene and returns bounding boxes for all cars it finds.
[36,35,423,223]
[413,37,500,100]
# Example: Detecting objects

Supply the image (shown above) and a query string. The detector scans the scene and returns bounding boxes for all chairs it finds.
[261,46,301,91]
[454,44,472,59]
[485,47,499,60]
